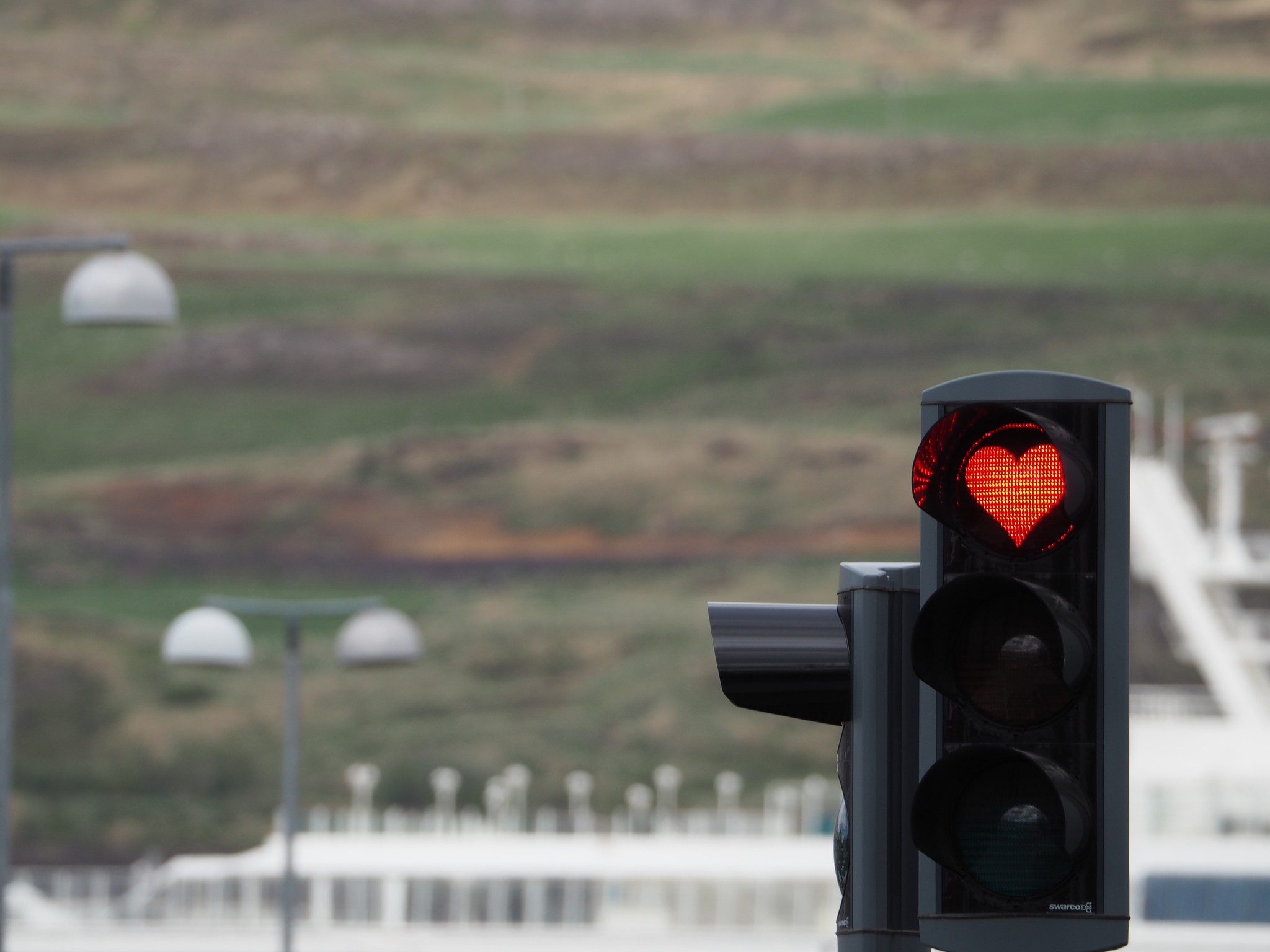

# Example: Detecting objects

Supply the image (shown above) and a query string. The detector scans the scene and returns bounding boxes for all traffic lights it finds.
[907,365,1134,951]
[706,554,920,952]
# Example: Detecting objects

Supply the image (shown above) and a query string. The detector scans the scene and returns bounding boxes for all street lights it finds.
[160,590,429,952]
[0,235,183,948]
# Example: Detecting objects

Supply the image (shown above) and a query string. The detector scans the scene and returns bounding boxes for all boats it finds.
[1136,366,1269,923]
